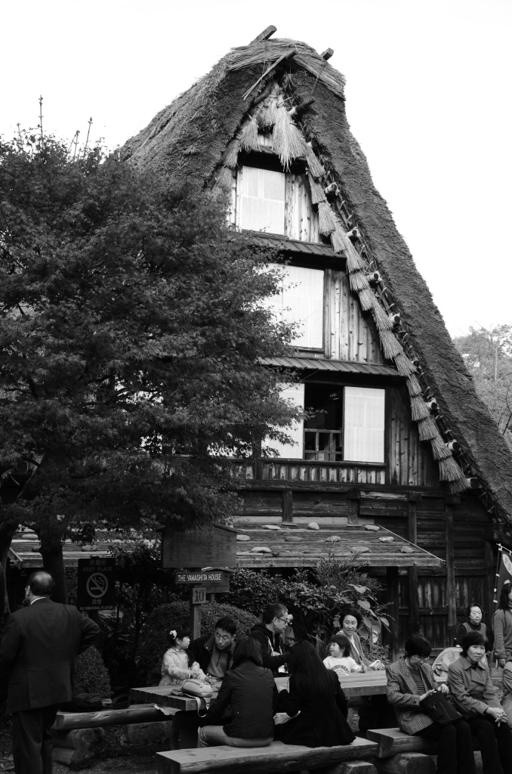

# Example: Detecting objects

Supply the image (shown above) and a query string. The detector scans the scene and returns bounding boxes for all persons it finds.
[159,603,372,748]
[386,583,512,773]
[0,570,102,774]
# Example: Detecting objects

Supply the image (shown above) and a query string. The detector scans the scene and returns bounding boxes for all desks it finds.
[128,667,450,750]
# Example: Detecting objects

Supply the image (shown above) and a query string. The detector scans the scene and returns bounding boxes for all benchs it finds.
[47,703,512,774]
